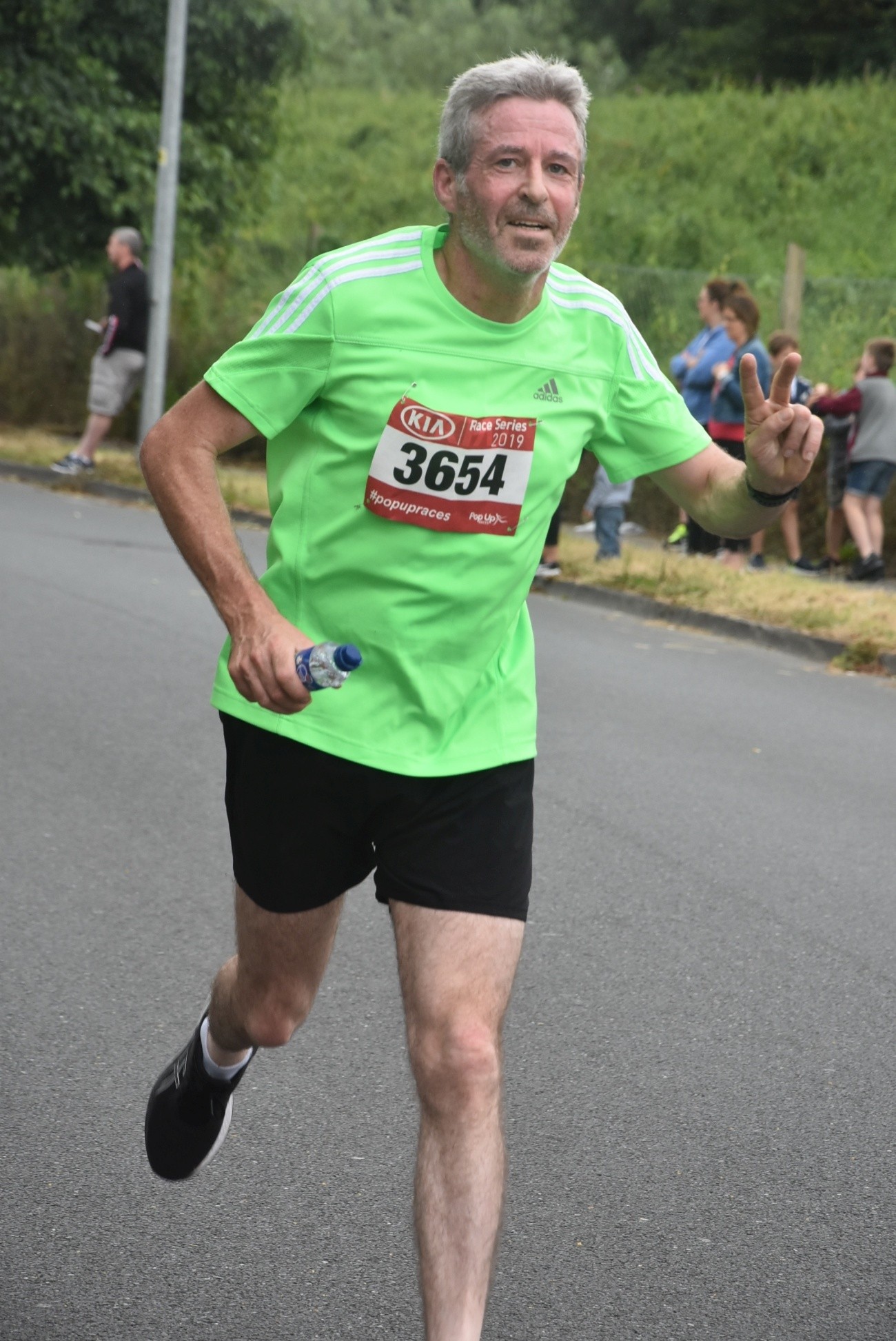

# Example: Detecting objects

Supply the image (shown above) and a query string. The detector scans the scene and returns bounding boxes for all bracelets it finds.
[744,470,798,508]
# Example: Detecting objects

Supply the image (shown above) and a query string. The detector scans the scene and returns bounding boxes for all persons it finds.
[535,276,896,584]
[140,54,825,1341]
[48,227,151,476]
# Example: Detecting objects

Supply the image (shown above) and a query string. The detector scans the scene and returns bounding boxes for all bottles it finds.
[296,638,361,692]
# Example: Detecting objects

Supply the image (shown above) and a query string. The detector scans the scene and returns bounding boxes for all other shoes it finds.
[50,455,98,477]
[748,553,765,569]
[534,561,562,578]
[784,554,845,576]
[666,522,688,543]
[847,554,887,582]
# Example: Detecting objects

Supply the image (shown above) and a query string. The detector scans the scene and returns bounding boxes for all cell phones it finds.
[85,319,103,332]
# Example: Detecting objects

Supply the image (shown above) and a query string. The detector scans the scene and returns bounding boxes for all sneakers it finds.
[145,1004,259,1180]
